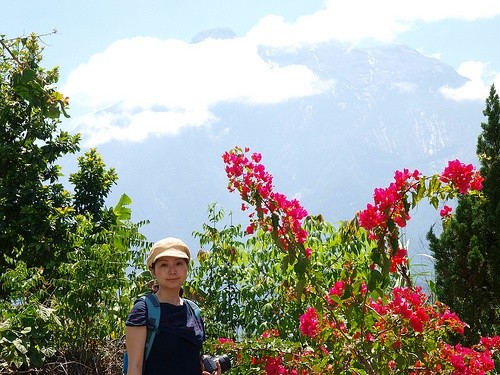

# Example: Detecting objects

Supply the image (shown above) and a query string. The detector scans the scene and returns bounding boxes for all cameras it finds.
[204,354,231,373]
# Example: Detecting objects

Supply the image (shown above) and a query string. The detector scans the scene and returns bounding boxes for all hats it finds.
[146,237,191,269]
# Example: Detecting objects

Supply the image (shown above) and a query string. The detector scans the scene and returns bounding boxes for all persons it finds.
[124,237,222,375]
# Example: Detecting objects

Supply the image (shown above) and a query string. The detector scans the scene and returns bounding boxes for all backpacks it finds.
[123,294,202,375]
